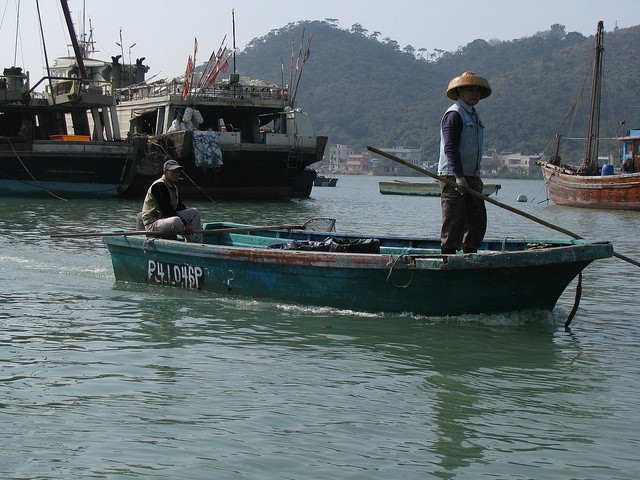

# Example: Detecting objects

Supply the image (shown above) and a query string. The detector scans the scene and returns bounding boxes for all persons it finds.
[437,71,491,255]
[142,160,203,244]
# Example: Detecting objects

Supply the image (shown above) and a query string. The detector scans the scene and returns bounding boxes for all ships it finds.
[0,0,147,196]
[35,0,328,201]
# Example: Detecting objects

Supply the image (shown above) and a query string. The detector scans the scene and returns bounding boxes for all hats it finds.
[164,160,183,171]
[446,72,491,101]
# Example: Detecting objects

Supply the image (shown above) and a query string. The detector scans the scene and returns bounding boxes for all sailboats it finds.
[535,21,640,209]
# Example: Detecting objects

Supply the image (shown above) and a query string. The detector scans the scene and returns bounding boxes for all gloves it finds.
[454,177,469,196]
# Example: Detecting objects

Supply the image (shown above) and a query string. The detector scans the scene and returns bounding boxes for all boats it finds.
[314,175,338,187]
[102,222,615,314]
[379,180,502,196]
